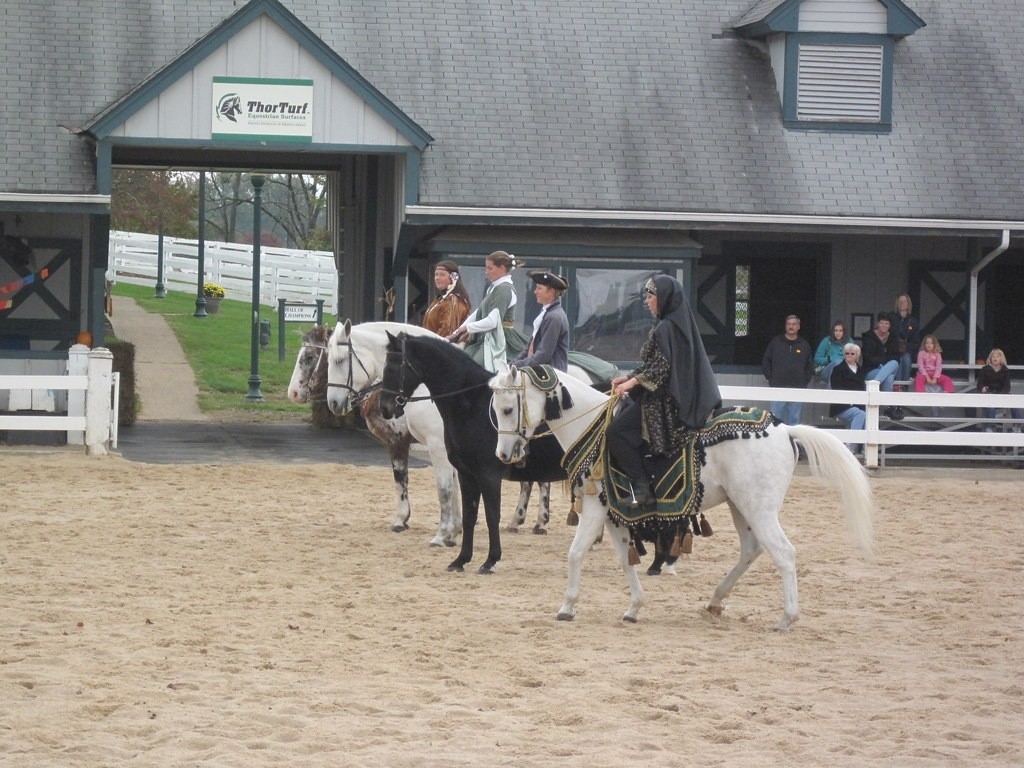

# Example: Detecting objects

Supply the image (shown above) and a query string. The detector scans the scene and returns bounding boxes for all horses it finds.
[285,318,879,632]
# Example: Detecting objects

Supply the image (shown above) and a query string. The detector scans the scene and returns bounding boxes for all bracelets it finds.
[453,328,462,337]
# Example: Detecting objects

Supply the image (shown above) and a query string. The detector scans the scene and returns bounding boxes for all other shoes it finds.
[855,450,865,458]
[887,407,905,420]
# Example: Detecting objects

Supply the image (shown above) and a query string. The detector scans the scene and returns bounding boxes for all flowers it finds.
[203,282,225,298]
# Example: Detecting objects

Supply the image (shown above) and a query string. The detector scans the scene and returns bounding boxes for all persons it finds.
[421,261,472,350]
[508,269,571,374]
[445,249,529,375]
[914,335,955,393]
[762,315,814,461]
[815,294,920,456]
[977,349,1024,433]
[604,274,722,509]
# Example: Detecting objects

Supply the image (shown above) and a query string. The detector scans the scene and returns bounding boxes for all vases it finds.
[205,298,222,313]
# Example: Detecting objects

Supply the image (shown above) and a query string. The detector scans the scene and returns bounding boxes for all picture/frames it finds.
[851,311,875,341]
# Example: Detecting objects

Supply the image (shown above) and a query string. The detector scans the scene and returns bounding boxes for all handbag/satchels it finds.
[814,365,825,376]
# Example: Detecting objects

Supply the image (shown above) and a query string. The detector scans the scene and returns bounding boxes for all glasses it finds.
[845,352,856,355]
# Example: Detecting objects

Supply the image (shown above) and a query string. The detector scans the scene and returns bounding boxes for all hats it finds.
[531,272,568,289]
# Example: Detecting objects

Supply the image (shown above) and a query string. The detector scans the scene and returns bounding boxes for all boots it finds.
[616,460,658,511]
